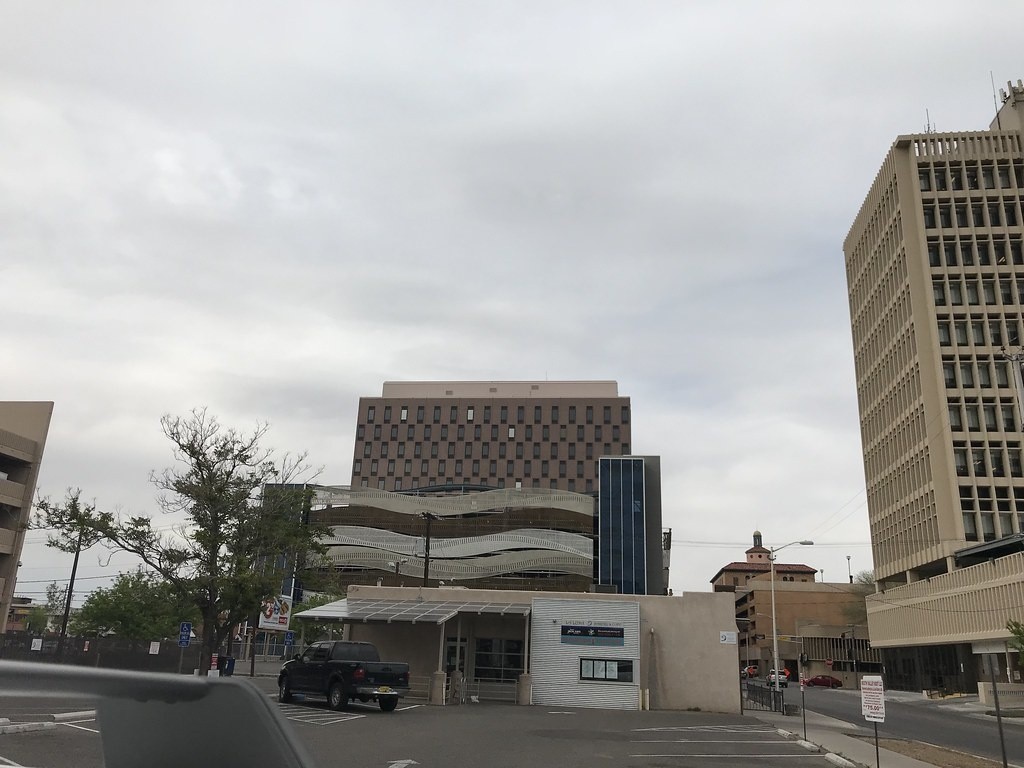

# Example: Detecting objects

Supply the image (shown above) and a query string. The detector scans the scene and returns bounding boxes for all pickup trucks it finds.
[278,640,412,712]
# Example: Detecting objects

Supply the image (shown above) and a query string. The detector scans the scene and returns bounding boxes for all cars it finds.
[804,675,843,689]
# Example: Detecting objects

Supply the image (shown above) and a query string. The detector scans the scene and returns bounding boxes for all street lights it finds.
[770,540,815,690]
[418,509,442,587]
[846,555,851,575]
[389,559,408,587]
[820,569,824,582]
[841,628,859,690]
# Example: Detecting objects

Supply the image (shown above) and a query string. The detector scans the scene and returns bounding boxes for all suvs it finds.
[766,669,788,688]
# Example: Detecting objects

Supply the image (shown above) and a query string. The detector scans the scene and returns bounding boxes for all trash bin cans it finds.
[220,656,235,677]
